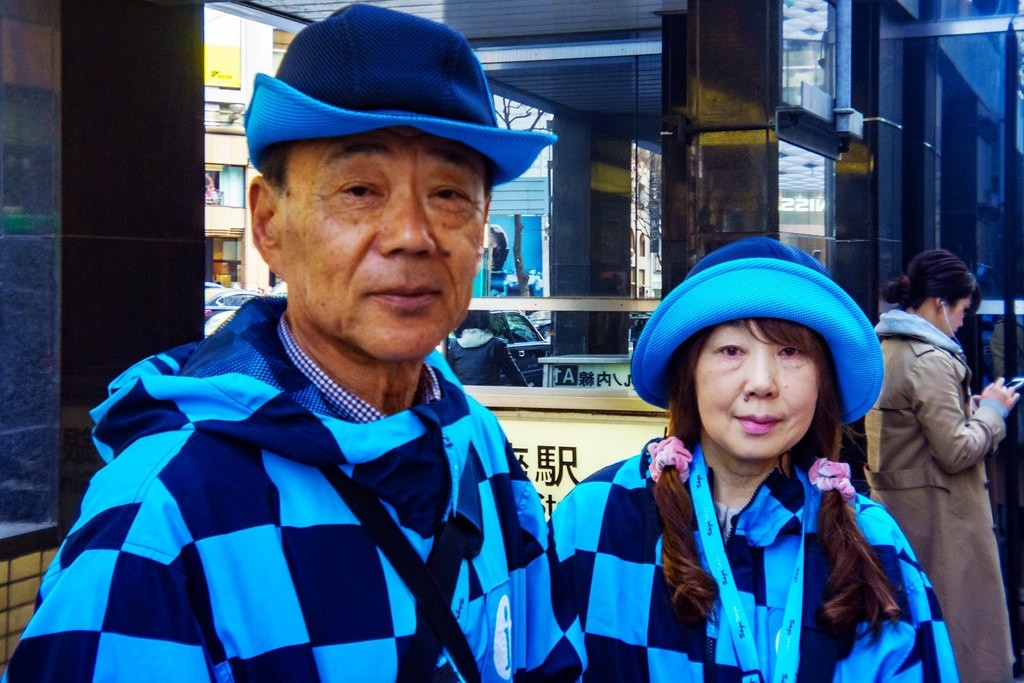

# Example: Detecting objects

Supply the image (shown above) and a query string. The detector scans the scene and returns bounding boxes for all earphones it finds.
[941,299,946,306]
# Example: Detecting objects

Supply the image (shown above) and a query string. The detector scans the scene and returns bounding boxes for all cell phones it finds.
[1003,378,1024,392]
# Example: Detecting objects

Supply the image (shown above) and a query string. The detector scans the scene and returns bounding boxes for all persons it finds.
[490,223,542,299]
[448,310,529,386]
[864,251,1020,683]
[991,315,1024,602]
[0,3,586,683]
[546,239,960,682]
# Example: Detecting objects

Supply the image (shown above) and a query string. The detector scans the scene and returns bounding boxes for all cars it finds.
[204,279,553,387]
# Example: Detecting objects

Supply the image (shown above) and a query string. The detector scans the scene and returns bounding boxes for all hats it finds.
[244,3,558,186]
[630,236,886,426]
